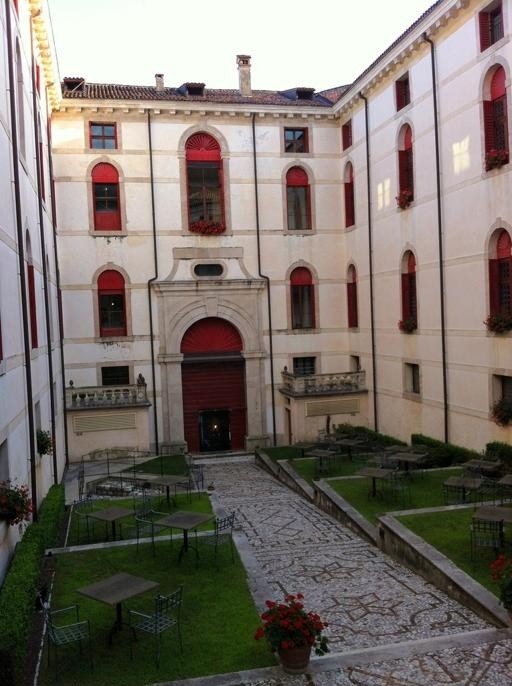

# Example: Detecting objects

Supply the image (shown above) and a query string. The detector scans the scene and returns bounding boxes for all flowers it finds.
[397,317,417,333]
[395,189,412,209]
[0,479,36,528]
[483,312,511,335]
[491,397,512,426]
[485,146,509,171]
[490,553,512,609]
[37,429,54,457]
[254,592,332,656]
[189,219,225,235]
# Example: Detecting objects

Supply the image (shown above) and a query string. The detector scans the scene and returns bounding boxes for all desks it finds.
[296,431,512,560]
[75,570,160,650]
[153,511,216,563]
[87,506,137,542]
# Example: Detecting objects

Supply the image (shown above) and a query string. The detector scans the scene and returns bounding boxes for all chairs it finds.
[73,498,109,545]
[119,464,204,508]
[128,584,184,668]
[43,605,94,680]
[196,510,236,572]
[134,500,171,557]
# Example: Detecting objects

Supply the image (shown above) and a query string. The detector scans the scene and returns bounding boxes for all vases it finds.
[277,643,311,675]
[507,608,512,619]
